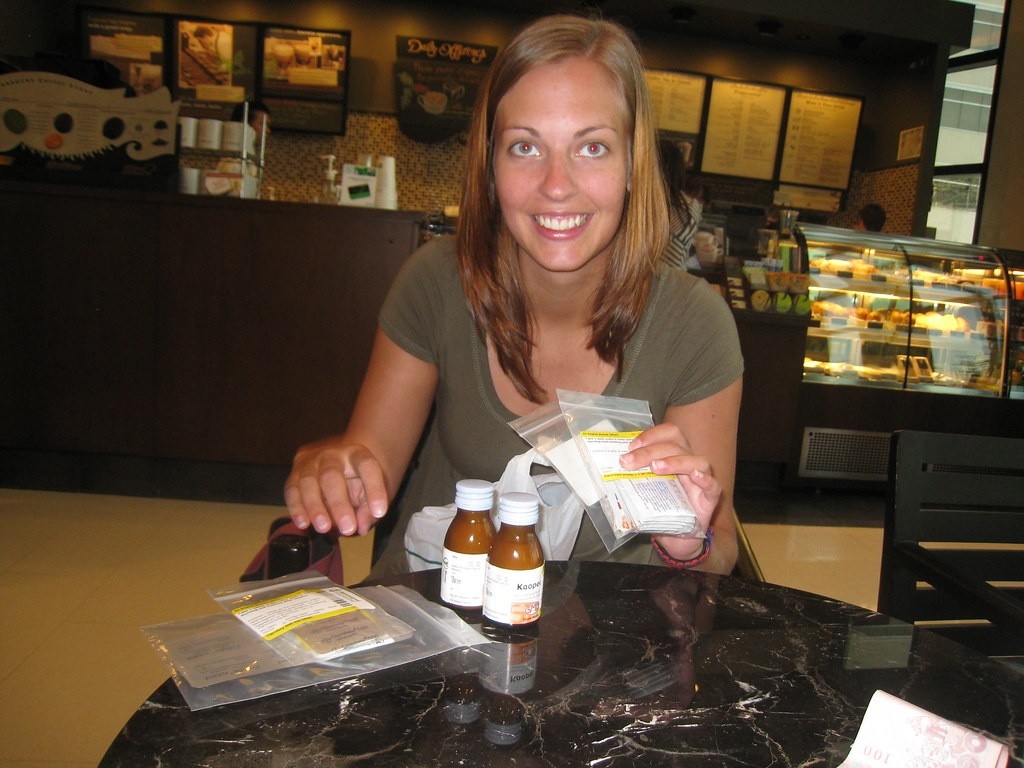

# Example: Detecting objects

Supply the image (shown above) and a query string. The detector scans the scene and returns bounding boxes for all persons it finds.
[282,16,744,583]
[660,140,702,271]
[859,203,886,232]
[194,26,233,60]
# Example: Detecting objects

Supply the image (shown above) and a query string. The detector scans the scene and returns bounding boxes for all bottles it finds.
[481,492,545,634]
[443,613,540,746]
[176,115,258,198]
[439,479,500,622]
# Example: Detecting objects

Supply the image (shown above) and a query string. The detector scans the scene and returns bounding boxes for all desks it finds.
[97,561,1023,768]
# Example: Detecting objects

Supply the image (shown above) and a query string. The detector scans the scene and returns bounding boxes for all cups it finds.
[780,210,799,235]
[373,155,399,211]
[757,229,778,256]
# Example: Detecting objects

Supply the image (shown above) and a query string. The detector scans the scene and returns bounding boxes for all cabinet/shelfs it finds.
[792,222,1024,496]
[179,99,271,199]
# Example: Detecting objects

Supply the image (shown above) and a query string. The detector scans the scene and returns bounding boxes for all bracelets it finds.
[650,529,715,569]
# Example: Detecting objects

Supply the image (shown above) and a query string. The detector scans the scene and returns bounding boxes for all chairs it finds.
[876,423,1024,664]
